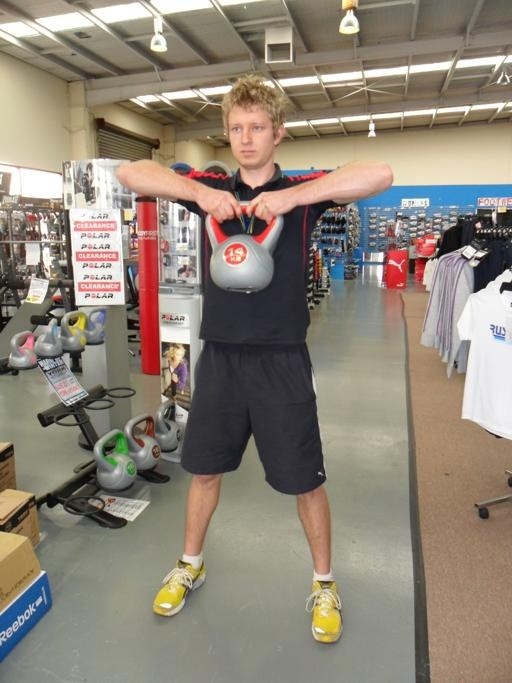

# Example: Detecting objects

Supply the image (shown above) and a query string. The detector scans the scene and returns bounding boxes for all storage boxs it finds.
[0,441,53,664]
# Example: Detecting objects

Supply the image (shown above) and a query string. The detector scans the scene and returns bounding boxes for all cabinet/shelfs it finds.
[0,207,70,280]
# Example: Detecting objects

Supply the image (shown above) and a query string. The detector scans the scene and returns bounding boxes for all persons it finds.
[109,74,399,646]
[158,342,188,403]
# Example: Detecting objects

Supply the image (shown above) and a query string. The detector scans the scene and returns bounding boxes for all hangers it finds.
[439,223,512,268]
[468,265,512,300]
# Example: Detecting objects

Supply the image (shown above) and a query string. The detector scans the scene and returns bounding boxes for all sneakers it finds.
[305,580,343,644]
[153,560,206,617]
